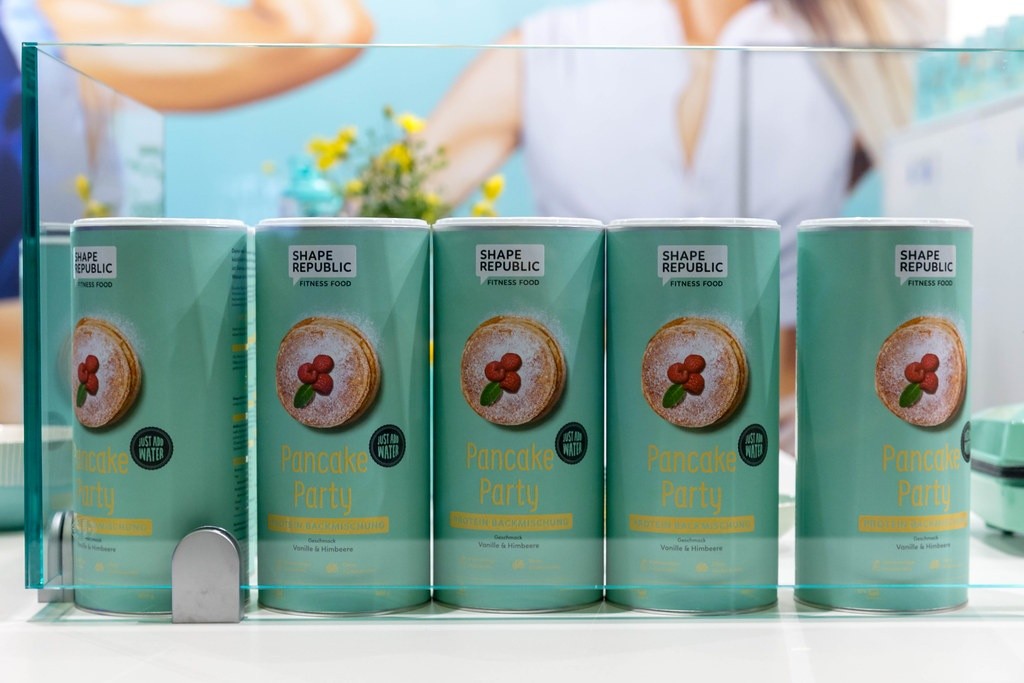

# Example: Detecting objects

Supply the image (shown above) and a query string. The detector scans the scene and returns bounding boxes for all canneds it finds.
[33,216,974,618]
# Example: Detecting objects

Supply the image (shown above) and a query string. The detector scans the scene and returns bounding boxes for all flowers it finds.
[308,107,506,226]
